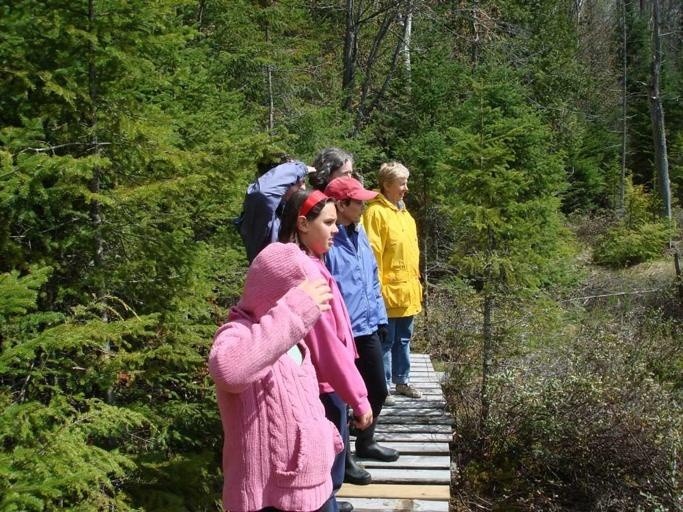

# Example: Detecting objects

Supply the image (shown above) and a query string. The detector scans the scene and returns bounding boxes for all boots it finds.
[343,426,372,485]
[356,415,399,461]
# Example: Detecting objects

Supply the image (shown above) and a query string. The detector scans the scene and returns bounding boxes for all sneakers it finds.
[396,383,422,398]
[384,392,395,406]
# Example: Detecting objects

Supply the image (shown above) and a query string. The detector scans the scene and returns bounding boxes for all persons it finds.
[360,161,423,408]
[206,241,346,512]
[237,151,319,261]
[307,143,363,439]
[323,174,402,485]
[276,188,376,511]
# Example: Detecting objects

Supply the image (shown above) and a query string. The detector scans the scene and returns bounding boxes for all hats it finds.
[324,177,380,201]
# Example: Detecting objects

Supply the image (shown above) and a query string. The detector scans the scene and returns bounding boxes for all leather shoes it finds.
[336,501,353,512]
